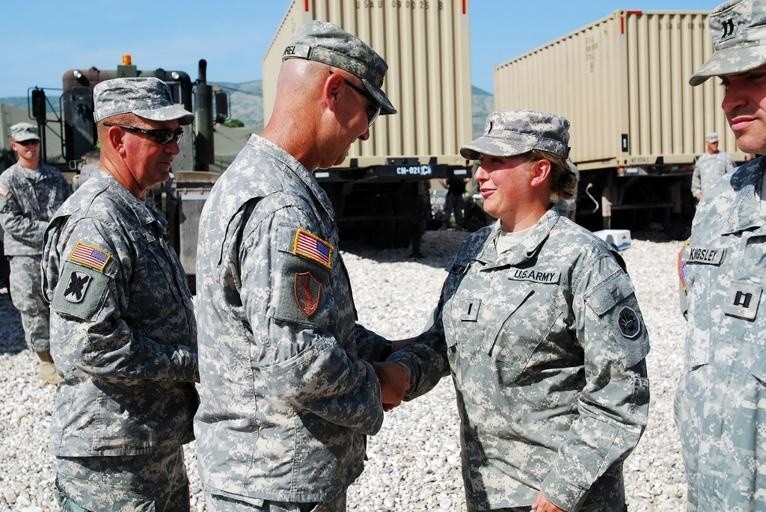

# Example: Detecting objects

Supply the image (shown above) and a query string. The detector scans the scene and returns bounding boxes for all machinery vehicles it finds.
[24,54,245,281]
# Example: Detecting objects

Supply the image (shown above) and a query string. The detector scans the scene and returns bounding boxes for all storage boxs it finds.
[592,228,632,252]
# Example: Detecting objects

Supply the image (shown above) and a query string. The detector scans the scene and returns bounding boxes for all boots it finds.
[36,351,57,385]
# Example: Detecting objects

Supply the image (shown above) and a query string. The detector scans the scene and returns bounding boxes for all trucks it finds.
[471,11,765,240]
[261,1,474,248]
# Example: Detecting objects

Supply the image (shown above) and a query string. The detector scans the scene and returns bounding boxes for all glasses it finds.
[103,121,185,146]
[16,139,40,146]
[329,69,382,127]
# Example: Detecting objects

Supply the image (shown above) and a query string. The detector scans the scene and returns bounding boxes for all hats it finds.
[687,0,766,88]
[91,75,197,126]
[705,132,719,144]
[458,108,572,160]
[9,122,41,142]
[280,20,400,115]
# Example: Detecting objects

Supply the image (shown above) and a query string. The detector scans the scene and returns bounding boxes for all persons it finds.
[691,133,734,201]
[675,0,766,512]
[383,109,650,512]
[0,123,72,385]
[40,77,200,512]
[192,20,417,512]
